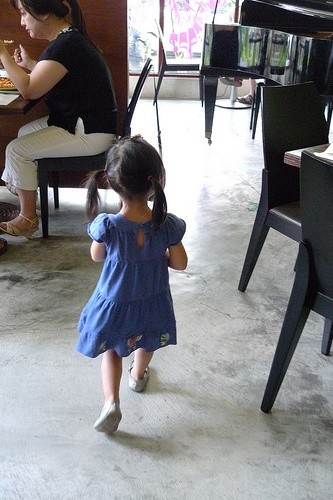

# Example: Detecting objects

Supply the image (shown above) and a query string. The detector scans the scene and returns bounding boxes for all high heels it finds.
[5,182,38,201]
[0,214,39,241]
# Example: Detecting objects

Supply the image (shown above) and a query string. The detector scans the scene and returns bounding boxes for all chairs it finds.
[238,82,330,292]
[260,150,333,415]
[37,56,152,239]
[153,18,204,106]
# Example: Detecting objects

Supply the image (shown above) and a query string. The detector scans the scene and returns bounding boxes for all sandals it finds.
[0,237,8,256]
[236,92,255,104]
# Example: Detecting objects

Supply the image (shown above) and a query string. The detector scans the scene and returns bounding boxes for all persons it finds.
[0,0,118,239]
[220,77,261,105]
[76,135,188,434]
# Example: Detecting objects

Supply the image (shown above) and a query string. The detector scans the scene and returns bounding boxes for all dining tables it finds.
[0,67,41,186]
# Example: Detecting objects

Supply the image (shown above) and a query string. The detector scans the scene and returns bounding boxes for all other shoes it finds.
[127,359,150,393]
[94,400,123,434]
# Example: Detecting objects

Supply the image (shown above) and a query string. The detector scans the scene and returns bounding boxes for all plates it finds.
[0,76,18,90]
